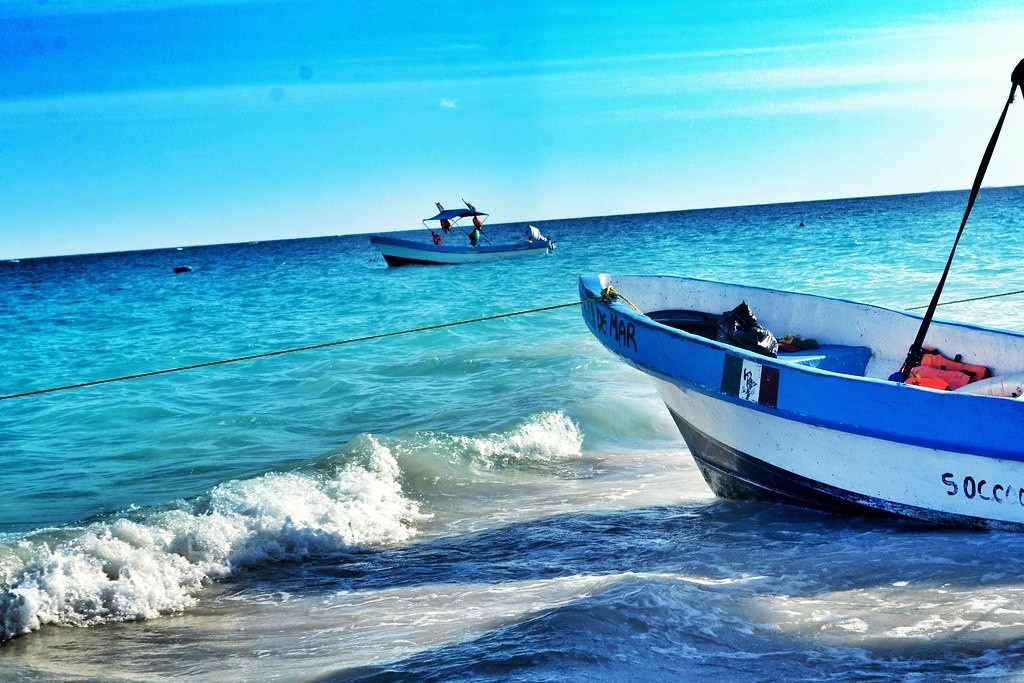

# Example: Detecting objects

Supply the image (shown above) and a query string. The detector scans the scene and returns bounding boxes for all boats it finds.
[370,197,557,267]
[577,271,1024,533]
